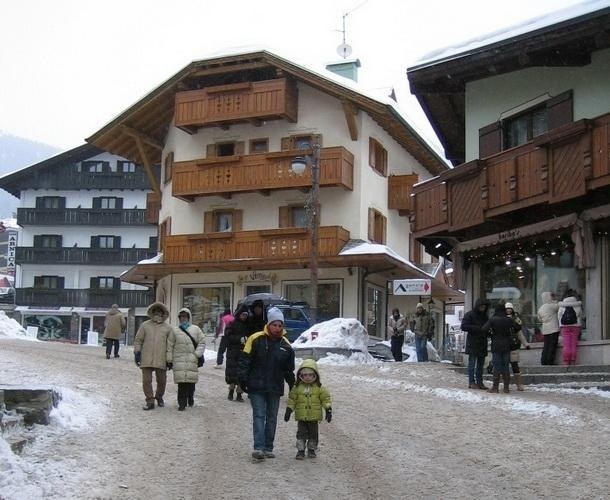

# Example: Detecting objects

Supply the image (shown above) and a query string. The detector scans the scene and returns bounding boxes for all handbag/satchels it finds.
[511,336,521,350]
[199,354,204,367]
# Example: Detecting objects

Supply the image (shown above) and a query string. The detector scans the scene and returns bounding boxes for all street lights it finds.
[291,141,322,328]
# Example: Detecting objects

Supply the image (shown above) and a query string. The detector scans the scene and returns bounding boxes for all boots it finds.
[489,369,524,392]
[228,390,244,401]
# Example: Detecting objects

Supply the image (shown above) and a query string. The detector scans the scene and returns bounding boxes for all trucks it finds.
[233,292,314,343]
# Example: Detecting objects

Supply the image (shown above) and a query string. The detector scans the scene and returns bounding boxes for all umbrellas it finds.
[241,293,286,321]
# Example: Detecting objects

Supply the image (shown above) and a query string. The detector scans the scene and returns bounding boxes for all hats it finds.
[505,302,513,308]
[417,303,423,309]
[267,307,284,328]
[392,308,399,316]
[303,368,313,375]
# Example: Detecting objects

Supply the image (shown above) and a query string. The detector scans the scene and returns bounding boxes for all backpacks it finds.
[561,307,577,324]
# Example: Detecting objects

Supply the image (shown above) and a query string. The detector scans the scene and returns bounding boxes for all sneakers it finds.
[252,451,275,459]
[114,354,119,357]
[296,450,304,458]
[178,397,194,411]
[542,360,575,365]
[215,363,223,369]
[144,397,164,409]
[308,449,315,457]
[107,353,110,359]
[469,384,488,389]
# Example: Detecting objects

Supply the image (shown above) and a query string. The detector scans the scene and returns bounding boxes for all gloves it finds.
[168,363,172,369]
[326,408,332,422]
[284,407,291,421]
[135,352,140,365]
[240,384,248,393]
[289,382,293,390]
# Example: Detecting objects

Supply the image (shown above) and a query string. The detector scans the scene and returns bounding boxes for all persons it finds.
[409,303,434,363]
[224,304,254,402]
[212,307,235,369]
[236,305,296,463]
[284,358,333,460]
[166,307,206,412]
[556,288,586,365]
[133,301,173,410]
[461,297,490,389]
[479,300,522,393]
[532,323,544,343]
[538,291,559,365]
[103,303,126,359]
[504,301,531,392]
[387,308,408,363]
[247,299,268,336]
